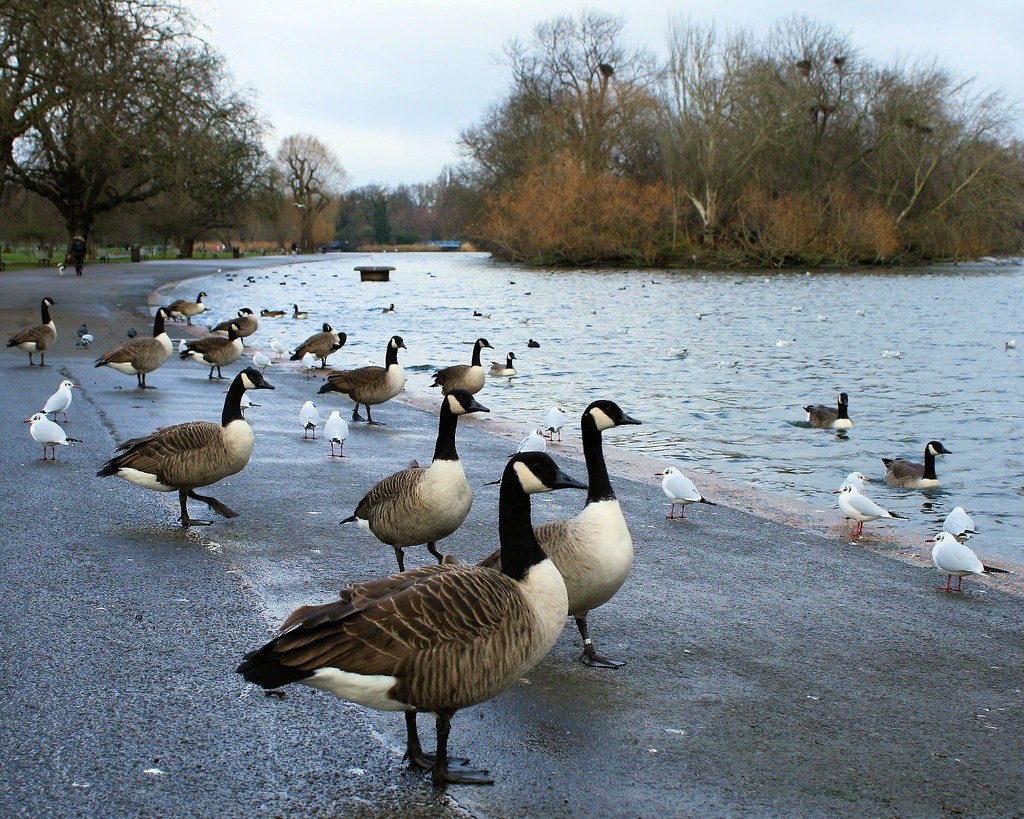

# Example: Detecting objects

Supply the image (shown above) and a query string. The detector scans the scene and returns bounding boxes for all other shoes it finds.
[77,268,82,276]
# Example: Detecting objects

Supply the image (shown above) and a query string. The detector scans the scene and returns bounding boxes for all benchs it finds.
[97,251,111,263]
[0,261,13,270]
[139,248,149,260]
[34,252,51,267]
[174,248,188,259]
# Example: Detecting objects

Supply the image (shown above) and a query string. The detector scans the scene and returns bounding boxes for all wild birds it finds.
[12,258,1024,791]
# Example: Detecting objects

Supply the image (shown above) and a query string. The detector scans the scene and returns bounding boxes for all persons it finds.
[291,241,297,257]
[68,228,87,276]
